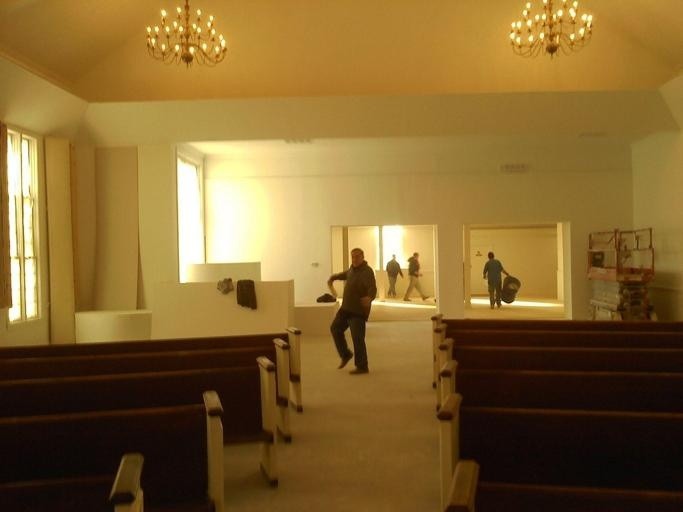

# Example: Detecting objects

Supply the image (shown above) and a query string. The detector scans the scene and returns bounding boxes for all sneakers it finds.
[340,351,353,368]
[350,368,369,374]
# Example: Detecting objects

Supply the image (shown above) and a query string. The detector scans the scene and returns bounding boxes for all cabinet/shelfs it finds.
[295,302,341,336]
[74,309,152,343]
[584,226,657,322]
[189,261,262,282]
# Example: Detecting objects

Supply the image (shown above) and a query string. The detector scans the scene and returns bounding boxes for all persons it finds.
[327,248,376,375]
[386,254,404,298]
[403,252,429,301]
[483,252,509,309]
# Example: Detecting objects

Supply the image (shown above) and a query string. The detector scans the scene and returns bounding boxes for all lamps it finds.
[144,0,228,69]
[508,0,596,61]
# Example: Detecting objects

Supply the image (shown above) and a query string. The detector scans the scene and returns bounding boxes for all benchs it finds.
[0,326,304,512]
[429,312,683,511]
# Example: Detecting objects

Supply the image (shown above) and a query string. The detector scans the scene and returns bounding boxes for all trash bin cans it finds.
[501,276,520,304]
